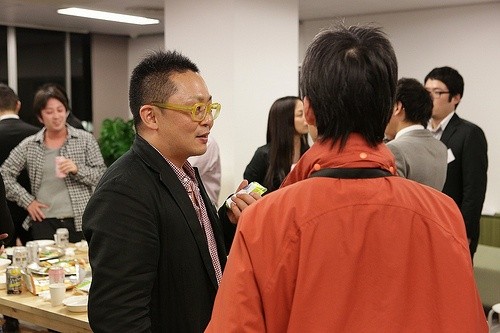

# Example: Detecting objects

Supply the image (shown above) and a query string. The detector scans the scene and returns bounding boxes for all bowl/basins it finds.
[62,295,88,313]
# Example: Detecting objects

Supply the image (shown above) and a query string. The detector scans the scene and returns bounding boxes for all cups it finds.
[49,283,66,307]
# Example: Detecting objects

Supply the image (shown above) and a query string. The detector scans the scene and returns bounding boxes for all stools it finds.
[487,303,500,333]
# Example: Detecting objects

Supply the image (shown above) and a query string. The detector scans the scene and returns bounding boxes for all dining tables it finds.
[0,242,95,333]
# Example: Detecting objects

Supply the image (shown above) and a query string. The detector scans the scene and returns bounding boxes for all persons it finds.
[81,51,263,332]
[380,78,449,194]
[0,86,108,243]
[49,83,88,131]
[185,134,220,210]
[0,84,39,247]
[244,96,312,197]
[425,67,488,270]
[204,26,490,333]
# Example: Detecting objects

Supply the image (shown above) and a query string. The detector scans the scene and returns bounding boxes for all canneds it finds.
[55,156,67,178]
[6,267,22,295]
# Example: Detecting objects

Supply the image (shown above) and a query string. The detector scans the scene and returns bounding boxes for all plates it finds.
[29,259,77,275]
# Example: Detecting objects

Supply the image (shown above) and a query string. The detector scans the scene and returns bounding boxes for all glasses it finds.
[425,91,450,98]
[140,103,221,122]
